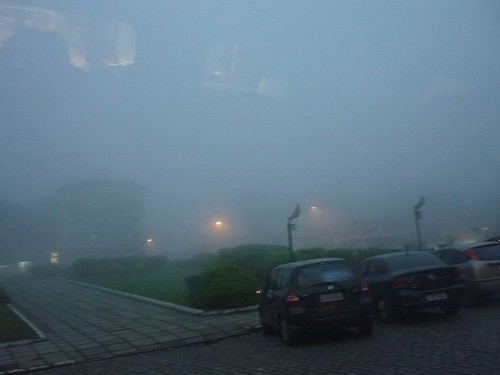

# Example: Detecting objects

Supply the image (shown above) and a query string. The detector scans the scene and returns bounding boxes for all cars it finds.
[260,257,374,344]
[433,242,499,306]
[356,251,465,324]
